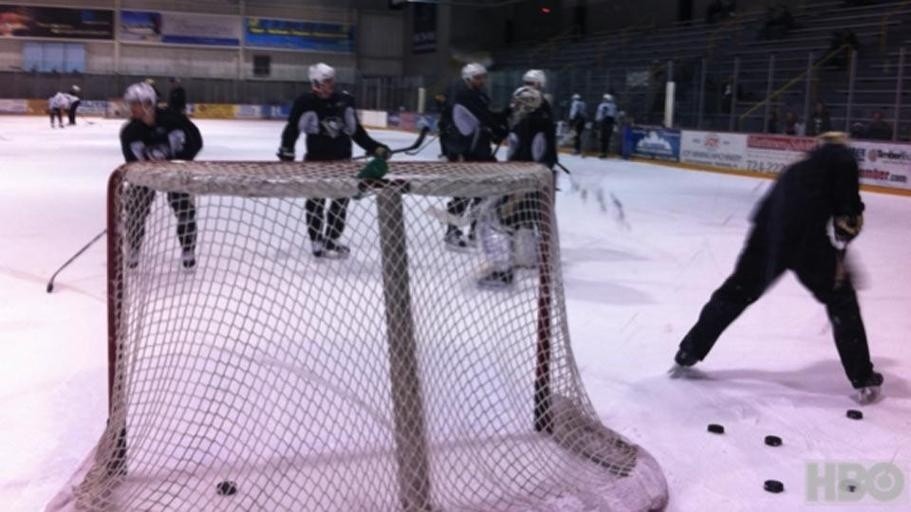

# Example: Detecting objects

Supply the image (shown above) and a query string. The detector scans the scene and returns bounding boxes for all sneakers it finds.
[484,272,512,282]
[311,242,321,256]
[444,232,465,247]
[183,250,194,266]
[127,249,137,268]
[468,231,475,238]
[851,372,882,388]
[675,348,696,366]
[323,237,349,252]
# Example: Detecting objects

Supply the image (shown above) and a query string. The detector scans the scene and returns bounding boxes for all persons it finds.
[49,91,64,126]
[118,81,203,265]
[569,95,587,153]
[477,87,556,289]
[809,100,829,133]
[438,63,502,251]
[595,94,616,158]
[505,70,552,112]
[165,77,186,113]
[63,93,78,126]
[784,108,807,137]
[277,63,390,255]
[666,132,884,403]
[865,111,892,142]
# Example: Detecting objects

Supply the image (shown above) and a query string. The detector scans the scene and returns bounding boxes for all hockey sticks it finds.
[352,125,430,161]
[76,110,94,125]
[47,227,110,292]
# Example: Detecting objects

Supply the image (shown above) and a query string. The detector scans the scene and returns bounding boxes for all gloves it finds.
[370,143,390,159]
[143,144,168,162]
[490,129,504,146]
[278,147,293,161]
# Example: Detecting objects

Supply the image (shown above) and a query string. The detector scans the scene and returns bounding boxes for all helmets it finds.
[144,78,154,85]
[571,94,580,99]
[513,86,541,112]
[123,82,156,107]
[308,62,334,84]
[603,93,611,100]
[818,131,843,146]
[522,69,544,86]
[72,85,79,91]
[461,62,486,81]
